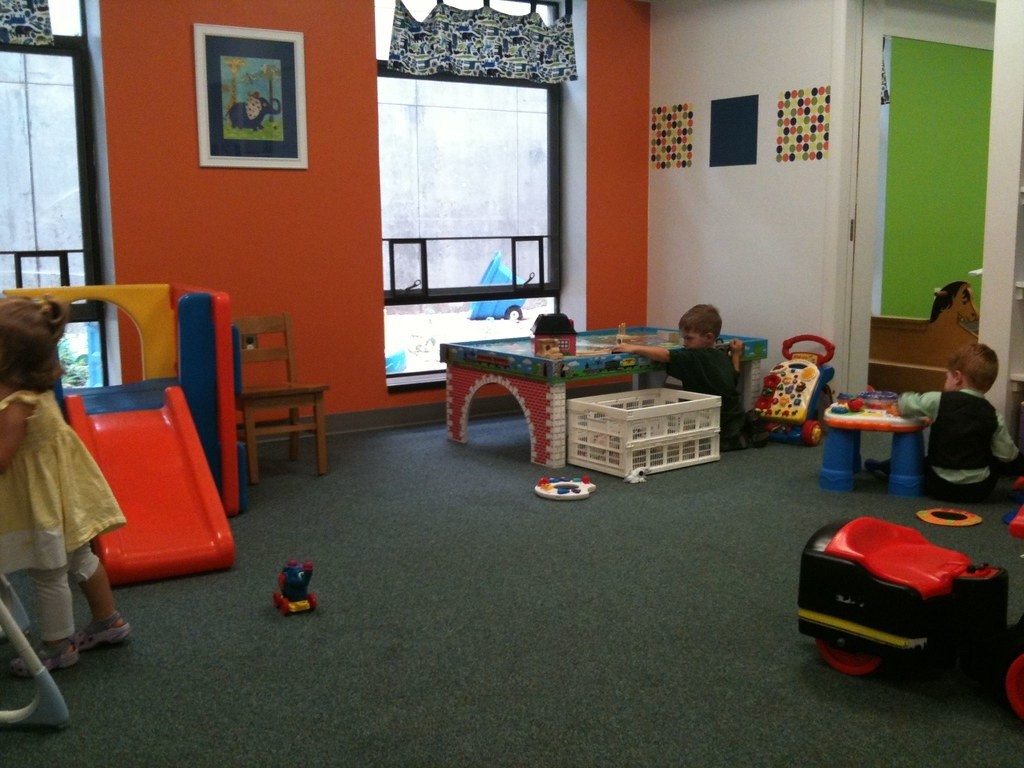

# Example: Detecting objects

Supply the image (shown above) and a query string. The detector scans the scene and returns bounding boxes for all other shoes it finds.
[11,651,79,675]
[75,622,131,651]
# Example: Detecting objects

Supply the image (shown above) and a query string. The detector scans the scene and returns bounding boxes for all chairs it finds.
[232,312,331,485]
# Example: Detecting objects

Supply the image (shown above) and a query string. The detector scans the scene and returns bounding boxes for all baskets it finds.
[565,387,722,475]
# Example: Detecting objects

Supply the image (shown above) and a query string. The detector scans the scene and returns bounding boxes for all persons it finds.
[611,305,749,452]
[866,344,1021,504]
[0,294,129,680]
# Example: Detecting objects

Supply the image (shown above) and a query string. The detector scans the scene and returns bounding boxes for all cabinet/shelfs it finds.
[818,400,932,498]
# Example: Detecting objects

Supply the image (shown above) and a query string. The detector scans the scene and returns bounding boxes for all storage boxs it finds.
[567,387,723,479]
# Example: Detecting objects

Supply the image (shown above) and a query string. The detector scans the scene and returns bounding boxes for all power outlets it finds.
[242,333,257,350]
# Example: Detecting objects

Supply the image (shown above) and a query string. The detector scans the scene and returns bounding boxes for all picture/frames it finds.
[193,22,309,171]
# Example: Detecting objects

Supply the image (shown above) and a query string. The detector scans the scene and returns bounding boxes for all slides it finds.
[65,386,237,589]
